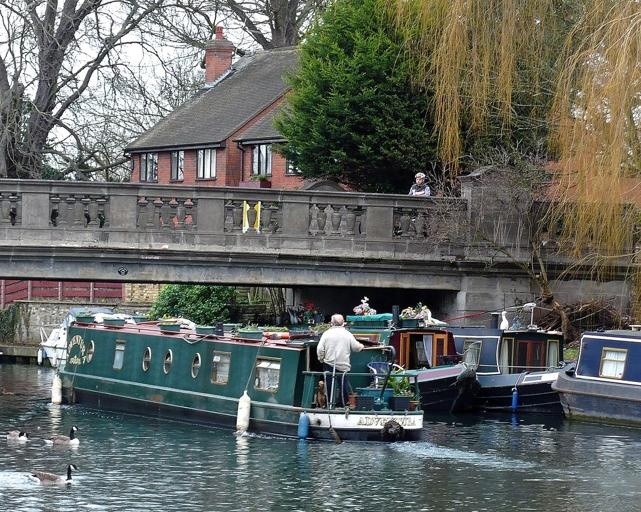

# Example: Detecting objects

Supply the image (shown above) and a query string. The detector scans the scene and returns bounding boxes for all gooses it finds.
[6,430,29,438]
[40,425,79,445]
[30,463,80,485]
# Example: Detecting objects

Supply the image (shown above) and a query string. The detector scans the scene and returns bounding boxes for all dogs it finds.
[313,380,326,408]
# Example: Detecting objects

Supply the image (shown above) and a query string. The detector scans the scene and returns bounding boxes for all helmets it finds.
[415,172,426,180]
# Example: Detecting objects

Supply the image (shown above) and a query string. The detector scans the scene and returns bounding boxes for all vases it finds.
[355,396,374,411]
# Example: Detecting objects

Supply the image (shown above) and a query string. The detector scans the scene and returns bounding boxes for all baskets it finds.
[348,394,375,411]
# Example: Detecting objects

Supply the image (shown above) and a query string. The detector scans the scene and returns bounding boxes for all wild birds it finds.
[500,309,509,330]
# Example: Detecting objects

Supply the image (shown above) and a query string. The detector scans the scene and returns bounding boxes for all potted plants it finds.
[380,363,415,410]
[408,395,423,411]
[347,380,358,408]
[74,311,330,341]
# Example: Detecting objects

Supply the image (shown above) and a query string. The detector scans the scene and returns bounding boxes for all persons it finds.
[317,313,366,407]
[408,172,431,196]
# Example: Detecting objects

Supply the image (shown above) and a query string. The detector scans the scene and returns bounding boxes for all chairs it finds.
[368,361,406,388]
